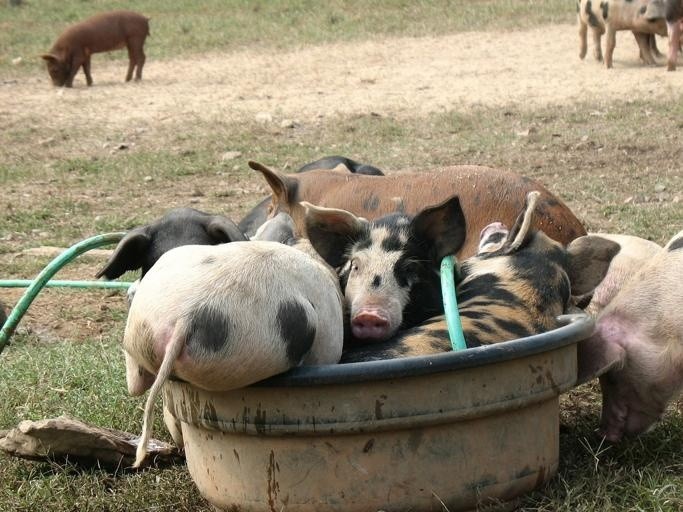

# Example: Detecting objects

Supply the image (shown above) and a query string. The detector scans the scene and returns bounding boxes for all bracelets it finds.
[153,315,596,502]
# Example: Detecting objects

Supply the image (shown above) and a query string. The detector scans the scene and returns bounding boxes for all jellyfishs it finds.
[574,0,683,72]
[40,11,153,89]
